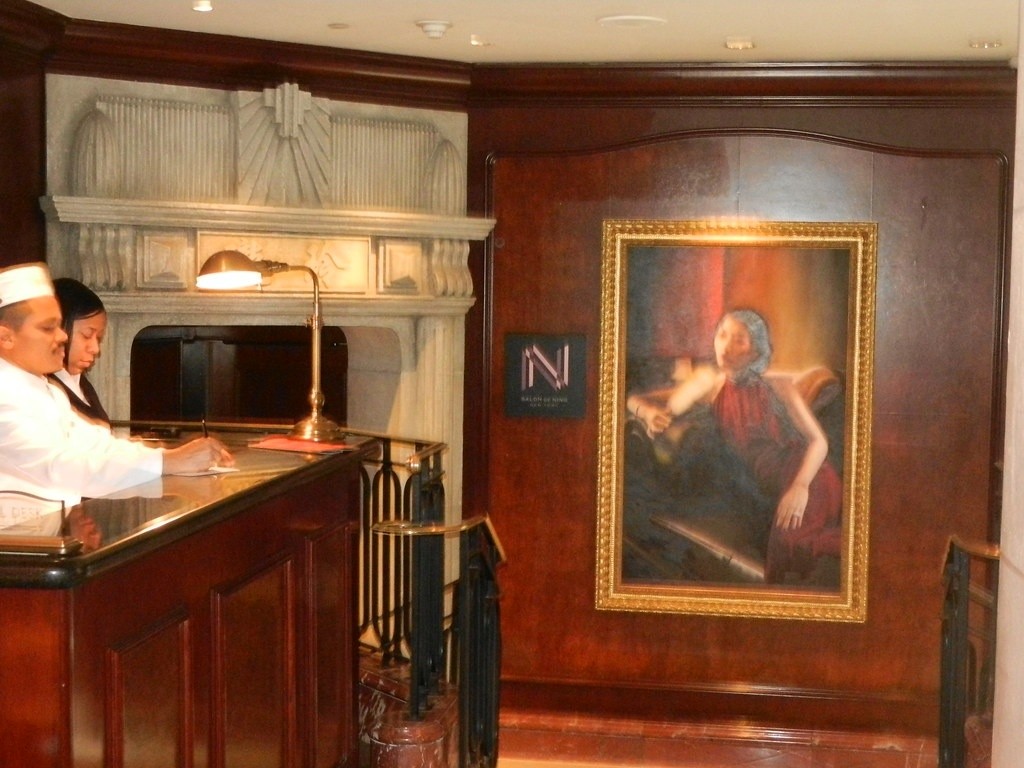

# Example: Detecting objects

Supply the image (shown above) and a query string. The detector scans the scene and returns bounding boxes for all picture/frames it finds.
[597,215,877,626]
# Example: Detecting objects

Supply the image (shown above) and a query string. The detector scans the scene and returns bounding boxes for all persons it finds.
[0,262,235,529]
[0,498,110,563]
[36,278,115,435]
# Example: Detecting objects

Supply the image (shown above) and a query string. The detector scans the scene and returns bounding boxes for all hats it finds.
[0,263,54,308]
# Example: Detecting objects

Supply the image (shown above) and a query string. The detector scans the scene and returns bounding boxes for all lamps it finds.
[192,249,350,443]
